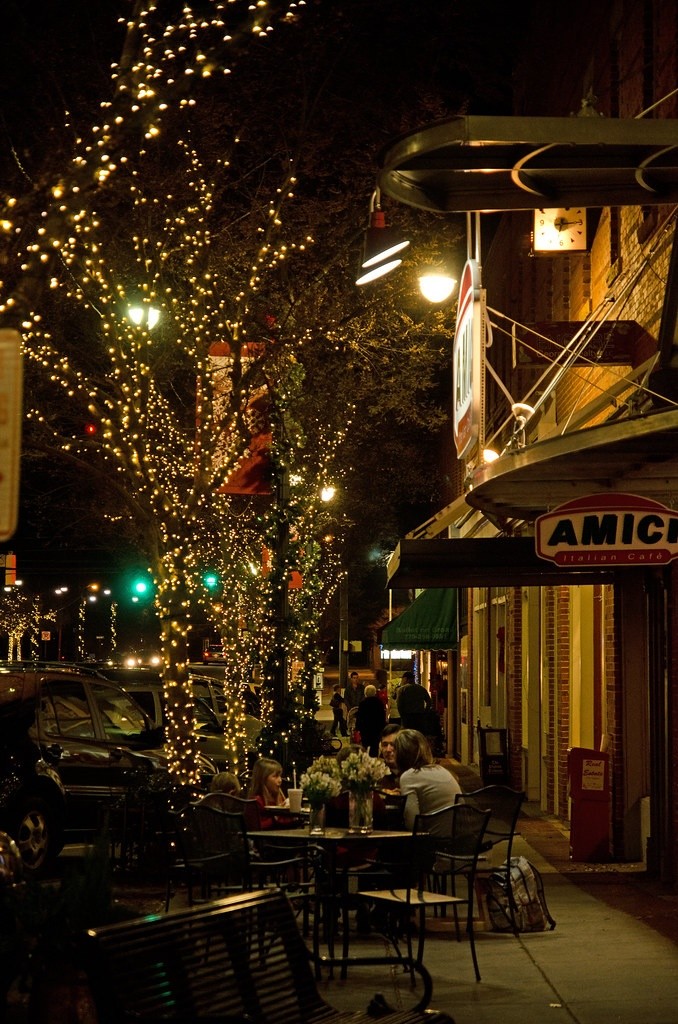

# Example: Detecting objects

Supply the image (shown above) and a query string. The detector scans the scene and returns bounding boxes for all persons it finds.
[345,667,448,797]
[330,684,351,737]
[196,772,268,885]
[244,757,318,915]
[372,728,466,935]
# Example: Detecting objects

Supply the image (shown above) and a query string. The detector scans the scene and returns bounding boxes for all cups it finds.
[288,789,303,813]
[600,734,613,753]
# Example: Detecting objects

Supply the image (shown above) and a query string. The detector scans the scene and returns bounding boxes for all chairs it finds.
[148,776,525,986]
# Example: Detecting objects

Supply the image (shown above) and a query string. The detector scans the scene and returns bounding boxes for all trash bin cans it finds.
[566,747,612,865]
[479,728,509,787]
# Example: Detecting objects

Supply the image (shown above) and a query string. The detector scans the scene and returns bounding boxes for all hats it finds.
[399,672,415,678]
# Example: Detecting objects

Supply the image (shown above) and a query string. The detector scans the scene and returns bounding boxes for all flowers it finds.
[343,746,391,791]
[301,755,342,800]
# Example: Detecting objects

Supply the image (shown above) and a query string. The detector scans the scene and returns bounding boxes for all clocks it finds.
[532,207,587,252]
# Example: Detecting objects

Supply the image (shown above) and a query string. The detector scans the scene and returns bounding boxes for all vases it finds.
[348,789,374,834]
[308,796,326,836]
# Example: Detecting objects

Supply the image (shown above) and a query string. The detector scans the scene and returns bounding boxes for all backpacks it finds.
[488,856,556,932]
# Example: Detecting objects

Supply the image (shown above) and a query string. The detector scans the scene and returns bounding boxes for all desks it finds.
[253,826,428,976]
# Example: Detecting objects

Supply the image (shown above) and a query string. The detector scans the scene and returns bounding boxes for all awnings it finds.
[377,590,460,651]
[384,536,678,590]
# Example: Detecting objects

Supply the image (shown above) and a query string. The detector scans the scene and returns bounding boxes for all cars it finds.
[0,660,219,843]
[106,664,270,766]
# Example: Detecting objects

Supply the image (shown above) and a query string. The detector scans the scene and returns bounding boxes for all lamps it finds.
[361,172,410,268]
[354,189,402,286]
[416,272,456,303]
[510,402,535,450]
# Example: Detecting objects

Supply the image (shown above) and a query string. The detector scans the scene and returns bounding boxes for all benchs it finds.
[88,881,455,1024]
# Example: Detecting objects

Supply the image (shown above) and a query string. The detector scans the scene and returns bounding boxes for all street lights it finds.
[318,480,355,680]
